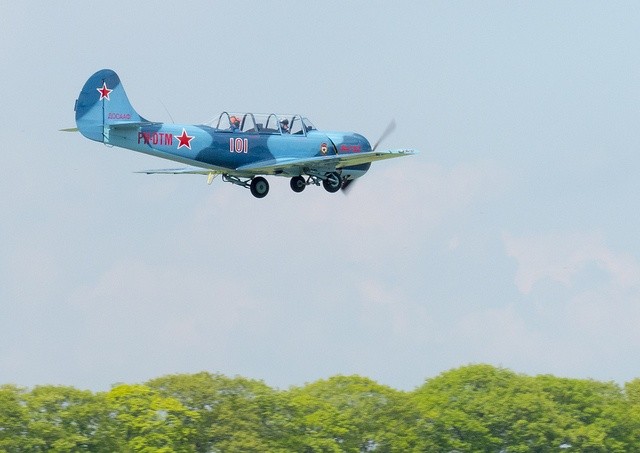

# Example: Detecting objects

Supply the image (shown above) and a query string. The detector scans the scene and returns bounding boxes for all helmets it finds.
[279,120,288,124]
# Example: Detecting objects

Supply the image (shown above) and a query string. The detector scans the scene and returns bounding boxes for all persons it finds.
[225,114,240,133]
[276,116,290,135]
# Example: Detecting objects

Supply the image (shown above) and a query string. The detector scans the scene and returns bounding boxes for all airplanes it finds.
[58,68,416,198]
